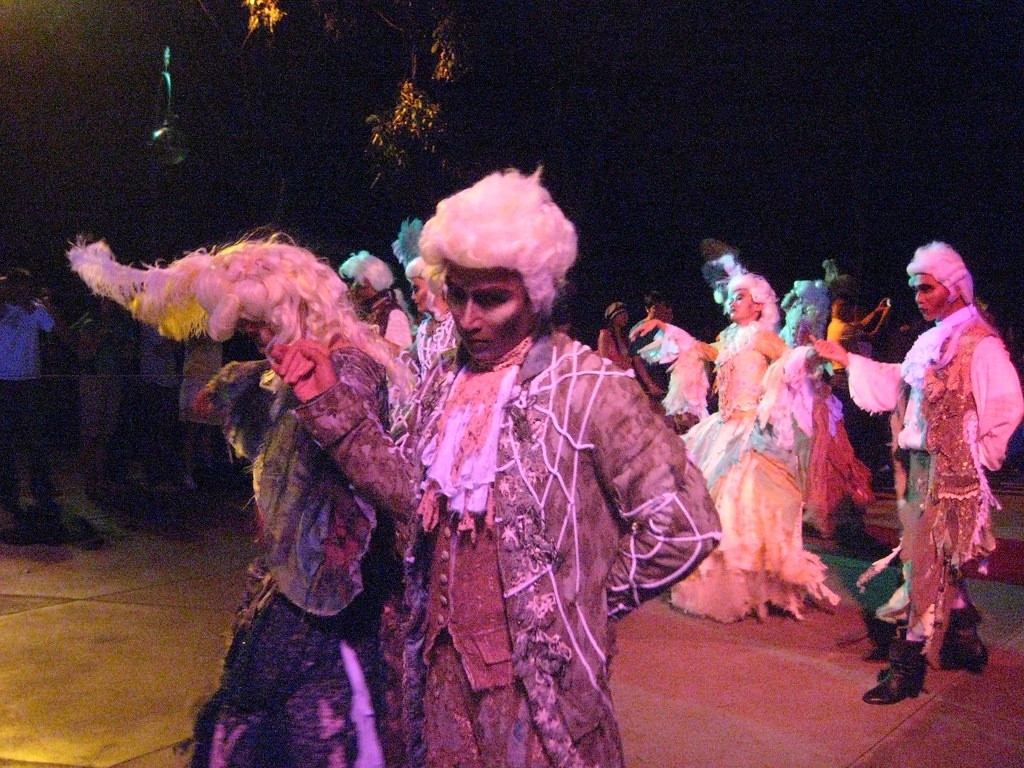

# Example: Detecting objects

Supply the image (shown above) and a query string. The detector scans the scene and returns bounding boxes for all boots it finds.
[864,639,927,705]
[939,607,988,672]
